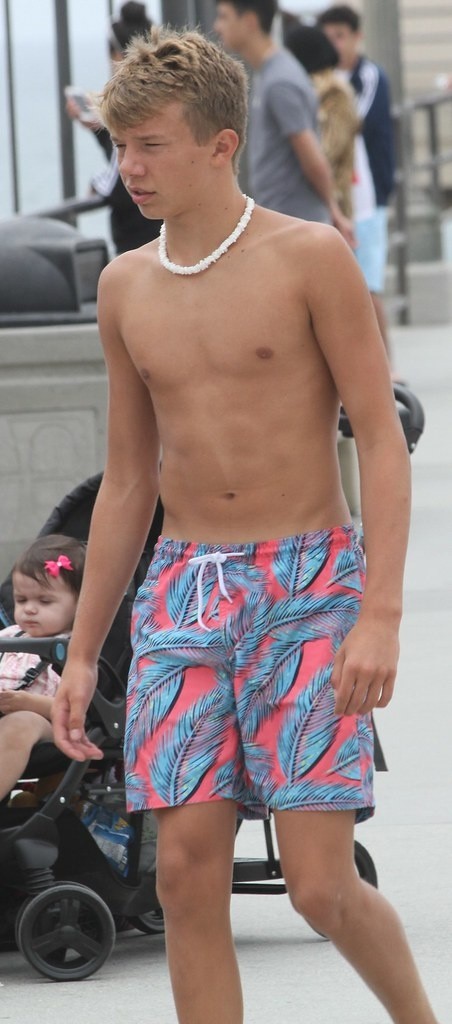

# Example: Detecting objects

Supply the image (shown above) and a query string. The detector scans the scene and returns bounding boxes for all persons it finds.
[67,0,165,256]
[319,6,396,356]
[50,27,438,1024]
[0,534,88,800]
[212,0,358,250]
[288,25,358,218]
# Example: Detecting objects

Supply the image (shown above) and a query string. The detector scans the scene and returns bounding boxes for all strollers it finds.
[0,382,425,982]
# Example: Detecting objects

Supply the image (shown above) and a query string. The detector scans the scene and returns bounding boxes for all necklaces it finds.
[158,194,255,275]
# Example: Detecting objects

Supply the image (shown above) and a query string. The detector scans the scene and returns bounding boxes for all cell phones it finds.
[64,85,94,122]
